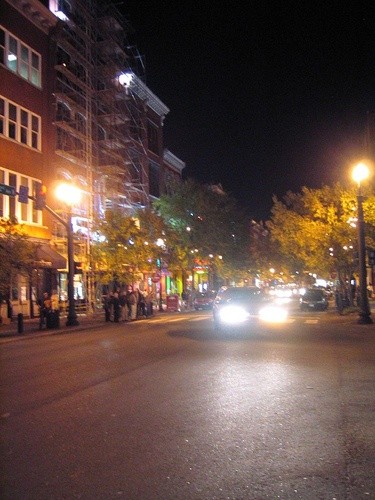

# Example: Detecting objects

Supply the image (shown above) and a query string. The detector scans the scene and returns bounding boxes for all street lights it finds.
[350,162,372,324]
[56,183,83,327]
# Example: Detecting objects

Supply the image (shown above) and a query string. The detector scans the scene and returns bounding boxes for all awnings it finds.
[0,238,66,270]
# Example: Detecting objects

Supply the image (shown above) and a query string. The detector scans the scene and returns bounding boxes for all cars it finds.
[194,292,213,311]
[211,286,279,336]
[299,288,329,311]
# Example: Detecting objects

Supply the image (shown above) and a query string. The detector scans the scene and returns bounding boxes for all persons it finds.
[103,286,149,322]
[366,282,374,299]
[37,304,46,330]
[355,285,361,304]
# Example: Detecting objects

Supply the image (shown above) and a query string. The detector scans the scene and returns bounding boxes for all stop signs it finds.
[150,273,161,283]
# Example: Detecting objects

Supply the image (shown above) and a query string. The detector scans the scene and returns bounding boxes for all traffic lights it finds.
[31,182,45,210]
